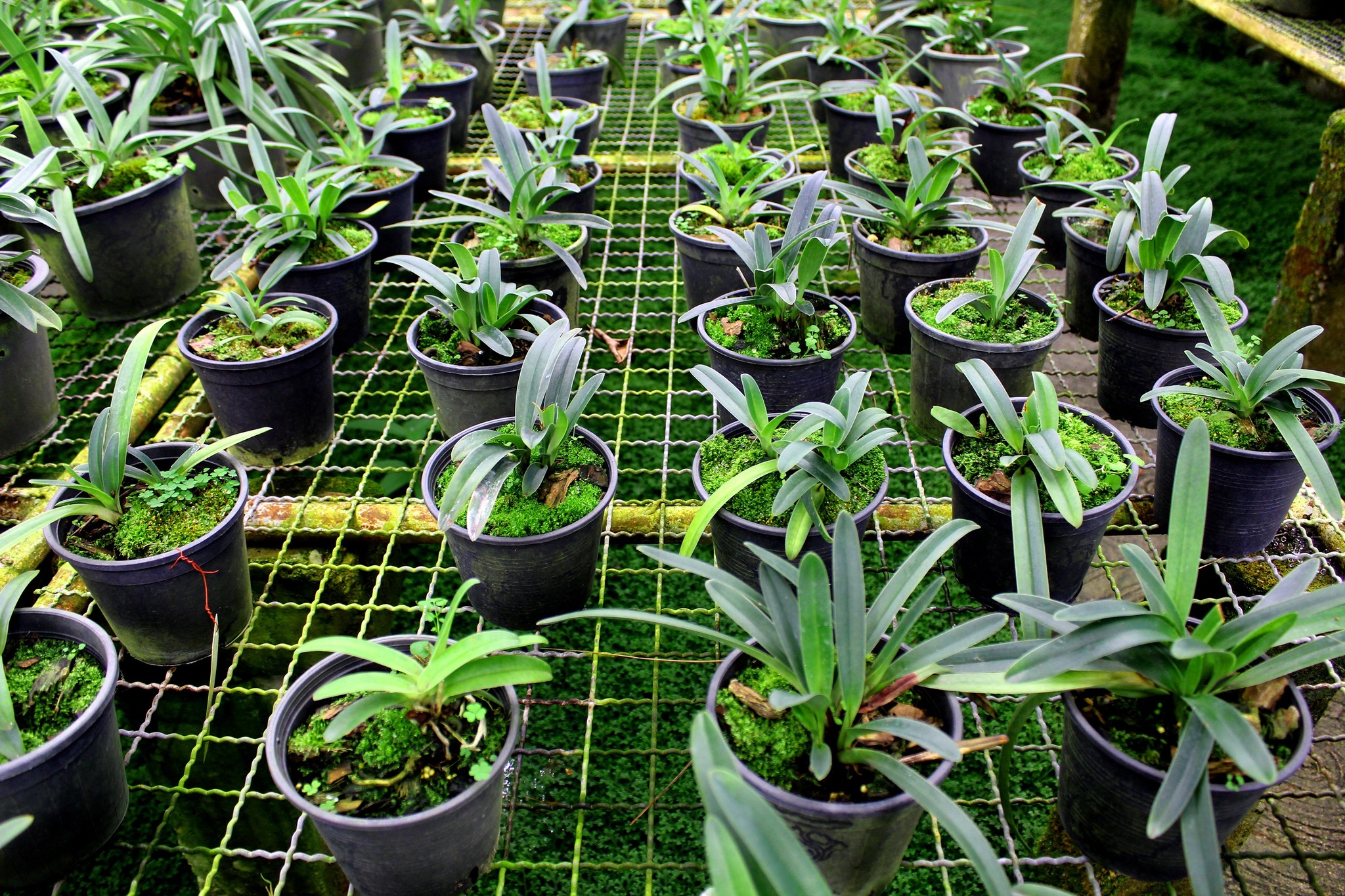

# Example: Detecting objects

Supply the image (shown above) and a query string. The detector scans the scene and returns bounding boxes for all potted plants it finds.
[0,0,1345,896]
